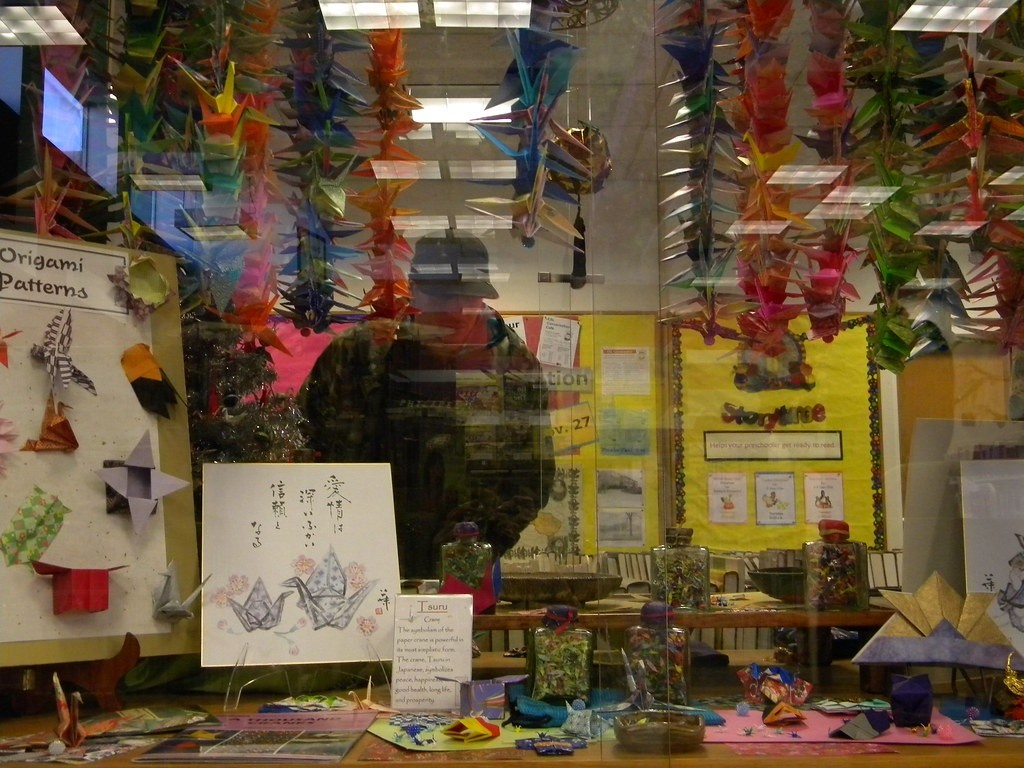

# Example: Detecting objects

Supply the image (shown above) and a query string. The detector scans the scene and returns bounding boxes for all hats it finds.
[408,227,499,299]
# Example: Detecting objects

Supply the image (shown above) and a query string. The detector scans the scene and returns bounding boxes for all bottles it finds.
[527,604,592,709]
[622,601,690,713]
[649,527,710,611]
[440,522,497,614]
[802,519,869,611]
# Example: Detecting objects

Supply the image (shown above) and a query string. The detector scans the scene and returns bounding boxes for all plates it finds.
[499,572,623,610]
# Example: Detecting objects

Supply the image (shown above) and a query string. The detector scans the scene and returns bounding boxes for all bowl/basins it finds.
[747,566,804,604]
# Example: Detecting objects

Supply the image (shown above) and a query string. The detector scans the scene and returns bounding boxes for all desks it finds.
[472,591,896,698]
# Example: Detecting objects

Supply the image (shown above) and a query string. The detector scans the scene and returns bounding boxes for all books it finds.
[131,710,380,761]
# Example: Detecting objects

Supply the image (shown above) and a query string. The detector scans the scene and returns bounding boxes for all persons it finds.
[294,225,555,582]
